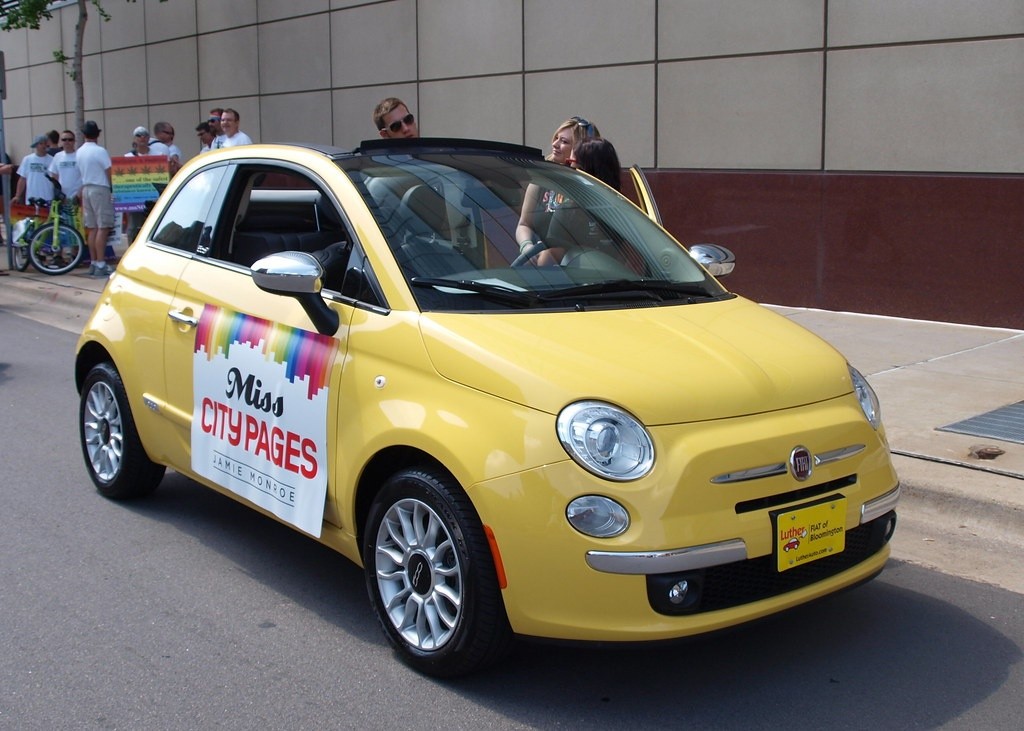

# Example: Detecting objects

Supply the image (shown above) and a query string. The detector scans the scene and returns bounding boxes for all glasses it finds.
[381,113,414,133]
[61,138,75,142]
[162,130,172,135]
[197,132,206,136]
[571,116,593,140]
[135,133,148,137]
[207,116,220,123]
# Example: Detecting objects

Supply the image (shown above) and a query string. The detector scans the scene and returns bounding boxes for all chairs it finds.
[297,195,347,253]
[230,234,296,272]
[394,186,478,279]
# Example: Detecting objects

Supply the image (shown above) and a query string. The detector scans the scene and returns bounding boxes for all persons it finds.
[515,116,600,254]
[373,98,453,243]
[0,162,14,277]
[9,127,64,270]
[111,121,184,248]
[74,120,116,279]
[536,136,628,268]
[47,130,83,268]
[194,107,253,156]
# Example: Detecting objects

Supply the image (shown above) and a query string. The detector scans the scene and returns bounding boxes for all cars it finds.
[75,137,900,682]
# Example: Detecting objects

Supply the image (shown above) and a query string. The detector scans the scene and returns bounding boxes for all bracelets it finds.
[520,240,533,253]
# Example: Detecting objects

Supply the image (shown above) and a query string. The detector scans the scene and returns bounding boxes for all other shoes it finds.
[53,256,67,267]
[94,261,115,276]
[88,264,96,275]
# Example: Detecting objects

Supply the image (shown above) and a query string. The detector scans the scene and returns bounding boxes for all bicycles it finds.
[11,171,85,276]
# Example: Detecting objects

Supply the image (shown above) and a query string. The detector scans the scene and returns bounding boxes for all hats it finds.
[133,126,149,136]
[30,134,48,147]
[80,121,102,134]
